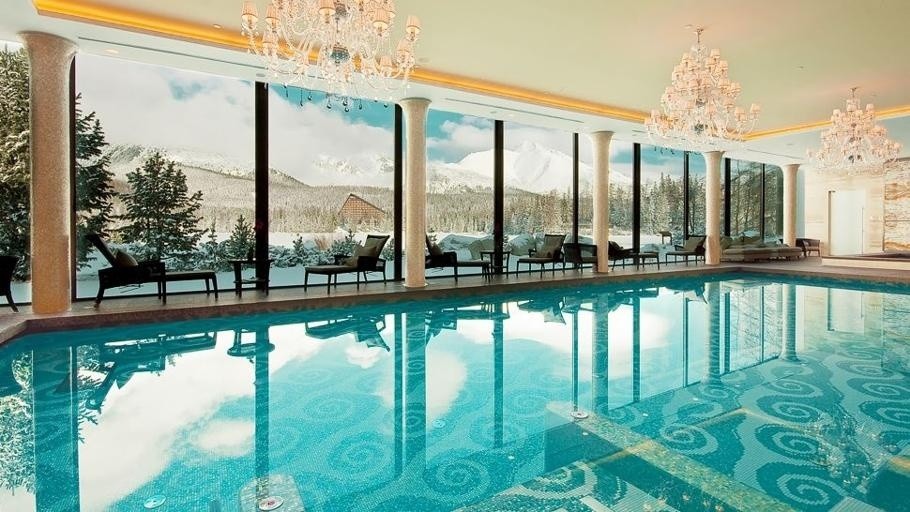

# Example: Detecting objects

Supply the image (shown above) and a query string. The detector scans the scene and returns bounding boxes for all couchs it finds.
[708,234,802,262]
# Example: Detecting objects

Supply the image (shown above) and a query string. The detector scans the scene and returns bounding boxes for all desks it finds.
[479,250,512,279]
[640,252,660,269]
[227,258,276,298]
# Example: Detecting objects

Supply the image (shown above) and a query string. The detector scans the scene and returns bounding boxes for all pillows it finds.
[115,248,139,267]
[341,244,377,266]
[535,245,556,257]
[720,237,729,250]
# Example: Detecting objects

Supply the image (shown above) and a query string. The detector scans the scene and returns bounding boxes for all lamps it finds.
[805,86,902,180]
[645,27,761,154]
[240,0,421,114]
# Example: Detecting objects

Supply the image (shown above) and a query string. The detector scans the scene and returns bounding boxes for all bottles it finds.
[246,247,254,260]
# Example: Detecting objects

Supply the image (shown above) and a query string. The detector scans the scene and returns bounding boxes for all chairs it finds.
[83,229,219,306]
[563,243,599,273]
[304,234,390,295]
[666,235,707,266]
[779,237,821,258]
[424,234,491,283]
[515,233,566,279]
[0,254,19,311]
[608,241,640,271]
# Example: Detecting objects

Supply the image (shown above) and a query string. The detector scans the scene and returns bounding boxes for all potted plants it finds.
[244,235,255,260]
